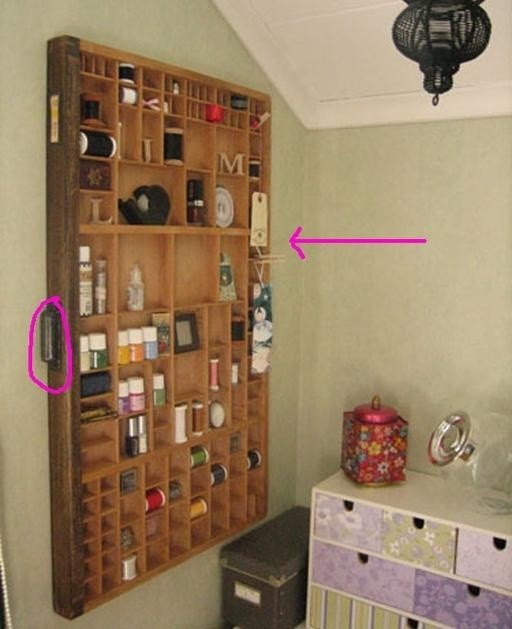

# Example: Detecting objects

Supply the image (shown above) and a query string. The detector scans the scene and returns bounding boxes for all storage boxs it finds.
[220,506,311,629]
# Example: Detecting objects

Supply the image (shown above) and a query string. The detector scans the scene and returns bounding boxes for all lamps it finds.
[392,0,492,106]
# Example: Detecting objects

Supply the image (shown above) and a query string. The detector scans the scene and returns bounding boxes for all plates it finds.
[214,188,234,227]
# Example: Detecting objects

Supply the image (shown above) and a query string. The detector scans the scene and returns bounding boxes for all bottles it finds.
[78,246,93,317]
[125,264,145,312]
[94,260,107,315]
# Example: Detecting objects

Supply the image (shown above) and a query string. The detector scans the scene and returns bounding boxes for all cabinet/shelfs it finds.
[306,466,512,629]
[46,37,274,620]
